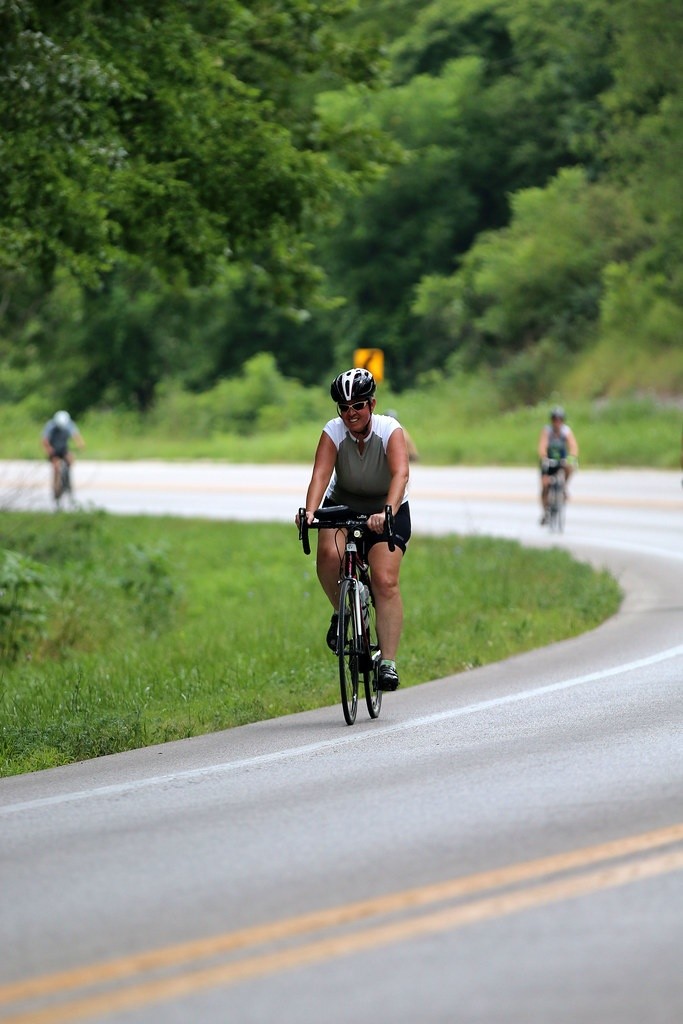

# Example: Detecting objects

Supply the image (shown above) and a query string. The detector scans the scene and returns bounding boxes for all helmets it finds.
[53,411,71,431]
[331,368,375,402]
[551,407,566,422]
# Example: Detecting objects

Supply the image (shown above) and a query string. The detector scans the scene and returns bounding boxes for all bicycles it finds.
[298,505,395,726]
[540,459,575,534]
[47,449,80,505]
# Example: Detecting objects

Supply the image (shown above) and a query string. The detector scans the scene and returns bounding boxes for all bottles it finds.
[357,579,369,629]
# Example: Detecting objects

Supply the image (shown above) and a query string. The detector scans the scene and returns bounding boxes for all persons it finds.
[383,409,418,461]
[42,412,83,498]
[295,368,411,689]
[536,406,578,525]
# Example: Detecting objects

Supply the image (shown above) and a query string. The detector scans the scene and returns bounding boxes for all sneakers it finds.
[377,666,399,691]
[326,612,349,653]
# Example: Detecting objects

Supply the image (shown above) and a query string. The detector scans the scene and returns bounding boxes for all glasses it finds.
[336,399,368,410]
